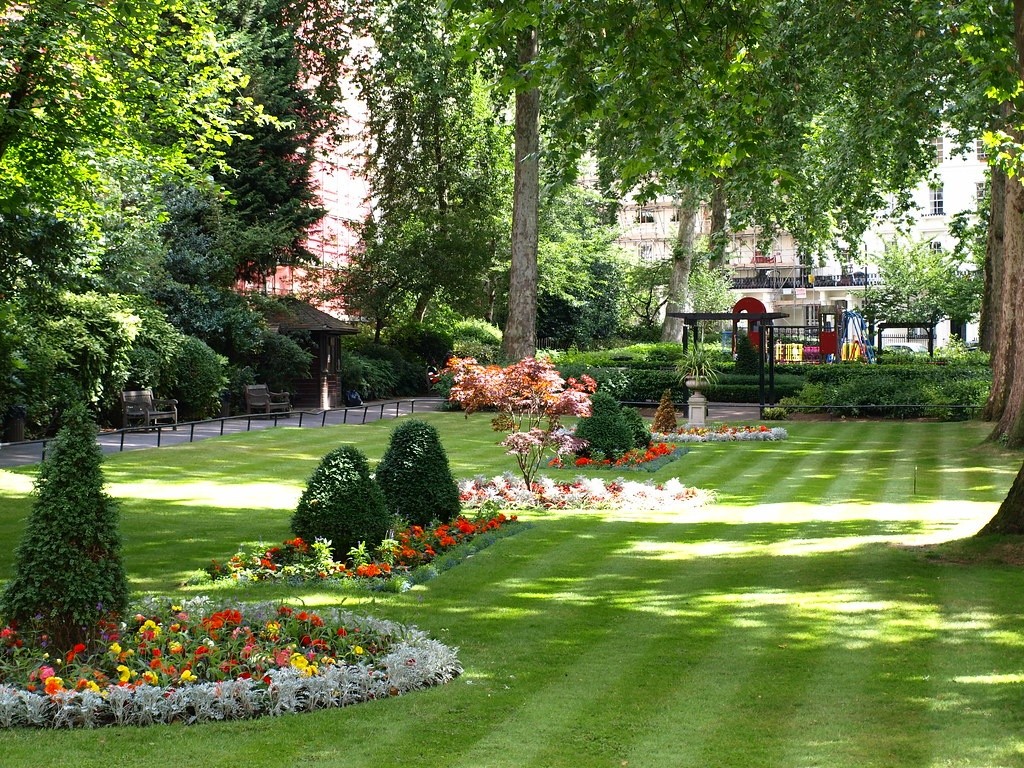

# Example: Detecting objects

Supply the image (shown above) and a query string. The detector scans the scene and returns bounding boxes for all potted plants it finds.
[673,344,726,389]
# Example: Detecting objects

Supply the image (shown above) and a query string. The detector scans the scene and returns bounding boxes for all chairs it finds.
[245,383,291,419]
[120,390,179,434]
